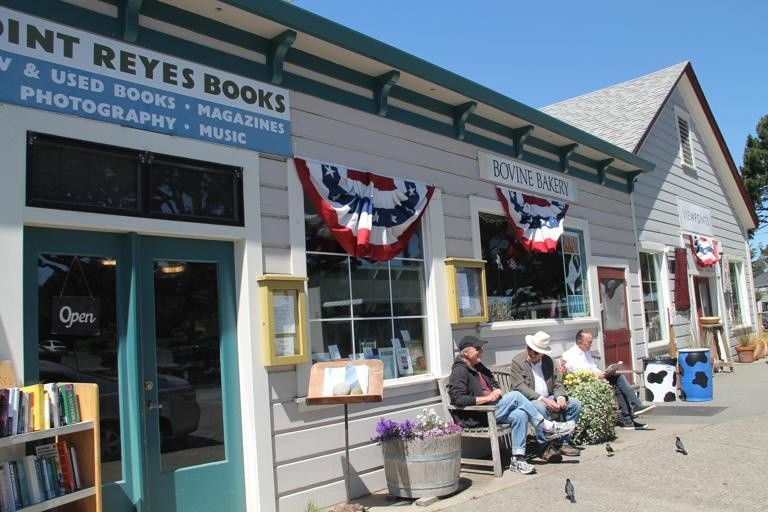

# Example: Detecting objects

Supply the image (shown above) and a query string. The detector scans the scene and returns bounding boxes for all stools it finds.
[698,322,734,377]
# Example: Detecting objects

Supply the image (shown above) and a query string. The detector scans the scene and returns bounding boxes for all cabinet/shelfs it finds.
[1,382,104,512]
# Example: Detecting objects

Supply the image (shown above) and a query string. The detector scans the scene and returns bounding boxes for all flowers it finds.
[558,359,619,445]
[368,407,464,446]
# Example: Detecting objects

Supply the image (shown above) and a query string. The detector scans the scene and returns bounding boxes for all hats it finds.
[525,331,552,353]
[459,336,488,350]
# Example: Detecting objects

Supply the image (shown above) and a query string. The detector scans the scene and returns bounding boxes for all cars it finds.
[33,329,220,462]
[508,296,569,317]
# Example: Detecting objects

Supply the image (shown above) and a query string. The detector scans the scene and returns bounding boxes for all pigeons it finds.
[564,478,577,503]
[605,444,615,454]
[675,436,688,456]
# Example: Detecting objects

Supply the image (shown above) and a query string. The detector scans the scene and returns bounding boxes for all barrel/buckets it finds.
[678,349,714,401]
[644,355,678,403]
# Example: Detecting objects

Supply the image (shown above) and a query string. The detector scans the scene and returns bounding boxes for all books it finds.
[0,382,83,512]
[602,359,624,378]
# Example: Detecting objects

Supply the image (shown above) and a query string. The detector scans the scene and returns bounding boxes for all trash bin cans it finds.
[643,348,713,402]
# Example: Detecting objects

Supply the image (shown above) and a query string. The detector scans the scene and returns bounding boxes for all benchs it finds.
[437,367,562,478]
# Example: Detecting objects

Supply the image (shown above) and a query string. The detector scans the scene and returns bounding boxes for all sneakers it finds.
[544,420,575,442]
[560,445,580,456]
[633,405,656,415]
[510,456,536,474]
[544,447,562,462]
[623,420,648,429]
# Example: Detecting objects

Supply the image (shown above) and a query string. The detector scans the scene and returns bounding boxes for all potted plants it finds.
[731,324,756,363]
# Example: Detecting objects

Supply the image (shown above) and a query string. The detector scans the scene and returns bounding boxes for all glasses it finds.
[526,346,539,355]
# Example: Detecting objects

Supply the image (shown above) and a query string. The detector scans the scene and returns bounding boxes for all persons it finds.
[561,331,656,432]
[447,333,578,473]
[566,255,583,296]
[508,330,583,463]
